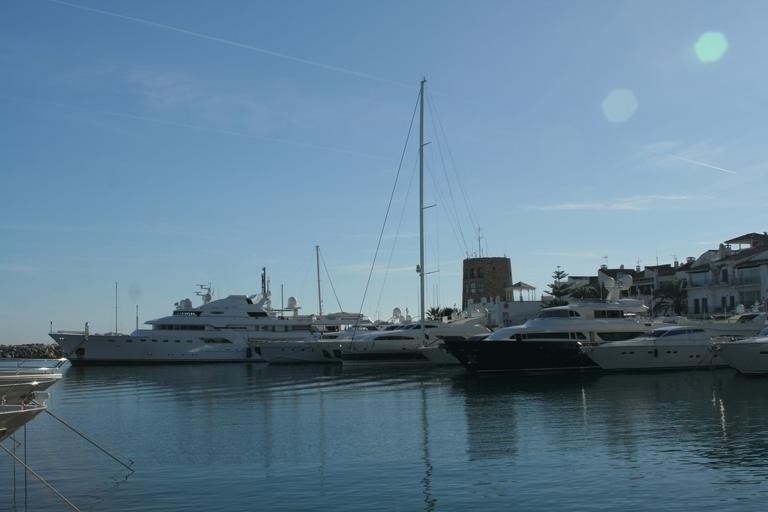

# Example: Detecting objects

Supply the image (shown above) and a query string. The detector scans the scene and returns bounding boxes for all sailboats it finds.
[244,75,493,367]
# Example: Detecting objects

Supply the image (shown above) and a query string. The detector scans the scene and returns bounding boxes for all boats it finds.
[0,349,76,448]
